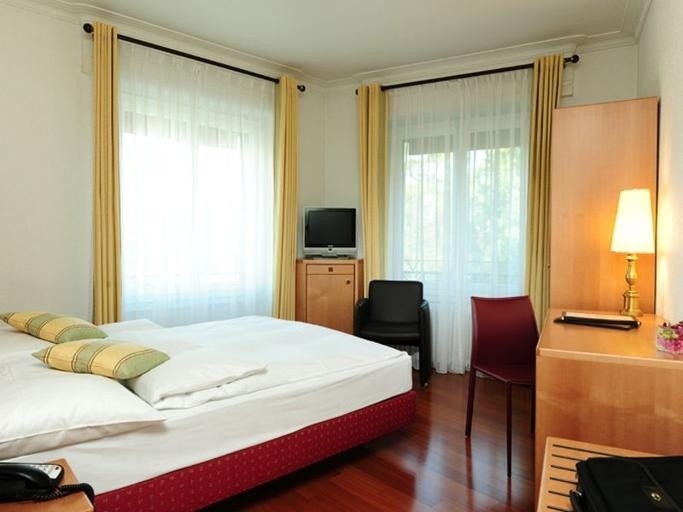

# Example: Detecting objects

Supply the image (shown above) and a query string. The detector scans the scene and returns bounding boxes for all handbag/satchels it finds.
[569,454,682,512]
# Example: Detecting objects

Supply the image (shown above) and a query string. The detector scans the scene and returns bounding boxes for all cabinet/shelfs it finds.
[296,256,362,335]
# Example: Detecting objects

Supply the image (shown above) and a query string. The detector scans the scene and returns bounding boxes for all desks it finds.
[533,307,683,511]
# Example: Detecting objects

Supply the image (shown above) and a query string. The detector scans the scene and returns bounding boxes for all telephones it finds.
[2,462,63,501]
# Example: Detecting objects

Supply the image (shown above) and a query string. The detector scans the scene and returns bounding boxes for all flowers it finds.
[655,320,682,357]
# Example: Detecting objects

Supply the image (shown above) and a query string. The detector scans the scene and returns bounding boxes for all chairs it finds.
[463,293,539,447]
[532,436,661,511]
[352,279,432,389]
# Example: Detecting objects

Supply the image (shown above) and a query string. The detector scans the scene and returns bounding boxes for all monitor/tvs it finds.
[301,206,359,261]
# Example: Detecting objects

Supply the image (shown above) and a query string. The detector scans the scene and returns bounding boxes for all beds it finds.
[0,314,418,512]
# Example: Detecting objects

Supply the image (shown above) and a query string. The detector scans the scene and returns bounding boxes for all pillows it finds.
[0,310,171,461]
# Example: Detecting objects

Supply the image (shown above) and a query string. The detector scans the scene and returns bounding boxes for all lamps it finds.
[609,188,656,317]
